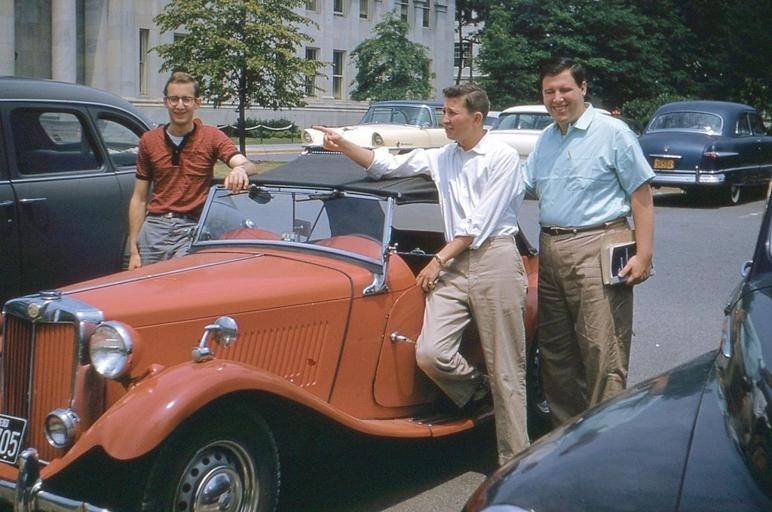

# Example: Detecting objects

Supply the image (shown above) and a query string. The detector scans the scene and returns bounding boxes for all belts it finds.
[148,211,198,220]
[540,223,606,237]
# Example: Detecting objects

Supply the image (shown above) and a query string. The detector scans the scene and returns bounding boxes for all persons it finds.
[308,82,534,469]
[126,71,258,271]
[520,55,657,430]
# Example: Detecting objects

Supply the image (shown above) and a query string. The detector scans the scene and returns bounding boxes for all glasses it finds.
[164,94,197,106]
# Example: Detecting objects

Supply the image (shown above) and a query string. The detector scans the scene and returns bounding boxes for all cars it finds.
[300,100,455,151]
[3,77,157,309]
[479,107,502,133]
[3,149,552,509]
[636,100,772,206]
[462,190,772,510]
[489,102,612,164]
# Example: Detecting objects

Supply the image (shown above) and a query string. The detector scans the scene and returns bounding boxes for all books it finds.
[607,240,655,286]
[601,228,656,287]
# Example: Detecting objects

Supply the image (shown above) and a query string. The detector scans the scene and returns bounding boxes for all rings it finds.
[428,281,433,288]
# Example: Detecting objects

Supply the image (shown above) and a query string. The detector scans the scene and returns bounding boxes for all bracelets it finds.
[432,252,446,269]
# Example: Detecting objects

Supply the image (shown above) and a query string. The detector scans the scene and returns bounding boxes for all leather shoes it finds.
[465,378,495,418]
